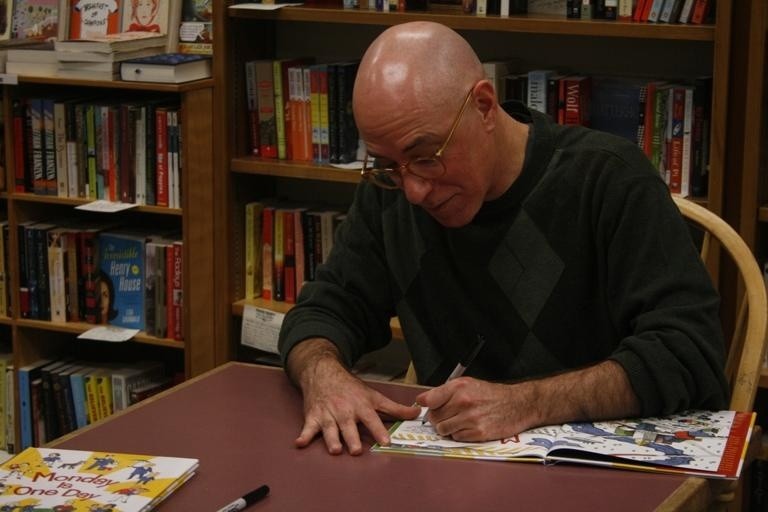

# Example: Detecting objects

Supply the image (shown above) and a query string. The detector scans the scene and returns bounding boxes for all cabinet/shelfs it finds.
[212,0,730,372]
[2,74,212,455]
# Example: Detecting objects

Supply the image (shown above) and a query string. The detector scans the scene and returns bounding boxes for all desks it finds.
[2,361,715,511]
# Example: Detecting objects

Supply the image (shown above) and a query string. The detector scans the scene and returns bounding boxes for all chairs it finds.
[669,193,768,511]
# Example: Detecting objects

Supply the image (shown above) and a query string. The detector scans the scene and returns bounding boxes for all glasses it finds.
[360,90,473,189]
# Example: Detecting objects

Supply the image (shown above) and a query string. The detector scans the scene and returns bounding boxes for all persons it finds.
[278,21,732,456]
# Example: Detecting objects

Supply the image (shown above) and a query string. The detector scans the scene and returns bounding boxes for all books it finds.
[0,446,199,512]
[1,2,213,455]
[368,402,758,481]
[242,1,713,306]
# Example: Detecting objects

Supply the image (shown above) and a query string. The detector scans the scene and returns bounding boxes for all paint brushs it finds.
[422,335,484,426]
[215,484,269,512]
